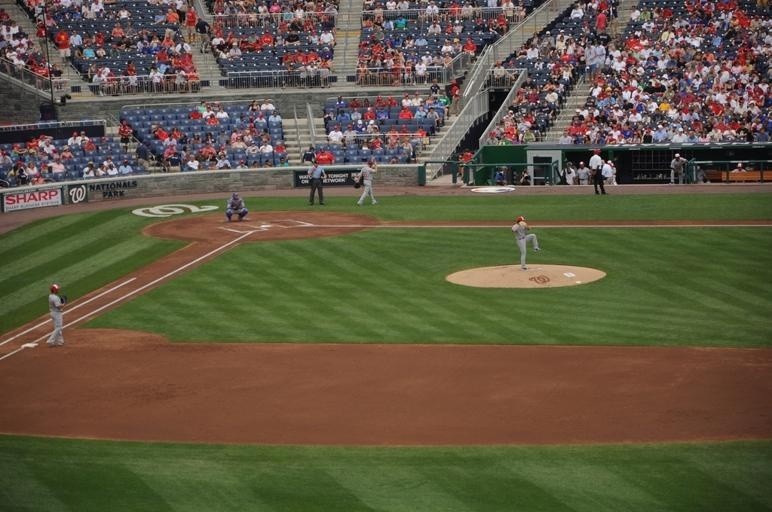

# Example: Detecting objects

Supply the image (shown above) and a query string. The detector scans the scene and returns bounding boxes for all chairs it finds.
[1,1,770,187]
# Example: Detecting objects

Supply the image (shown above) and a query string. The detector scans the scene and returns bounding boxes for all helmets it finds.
[518,217,525,221]
[51,284,59,292]
[368,160,373,165]
[232,192,239,199]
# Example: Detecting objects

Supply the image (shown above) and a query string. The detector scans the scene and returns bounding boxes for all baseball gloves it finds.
[61,295,67,304]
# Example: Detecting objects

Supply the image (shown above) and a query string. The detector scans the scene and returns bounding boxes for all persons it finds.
[512,216,541,271]
[226,192,248,222]
[46,283,66,346]
[357,159,378,206]
[308,159,327,206]
[1,0,464,187]
[588,148,610,195]
[455,0,771,185]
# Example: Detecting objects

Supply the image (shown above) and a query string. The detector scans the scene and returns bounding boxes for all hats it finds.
[314,160,320,165]
